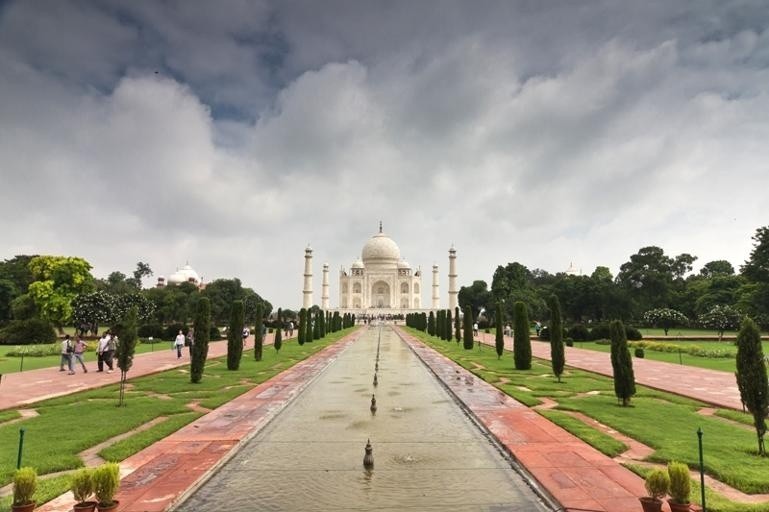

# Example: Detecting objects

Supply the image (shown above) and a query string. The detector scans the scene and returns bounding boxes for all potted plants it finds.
[70,462,119,512]
[639,460,693,512]
[11,466,37,512]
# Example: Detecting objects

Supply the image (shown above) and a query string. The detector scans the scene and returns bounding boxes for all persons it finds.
[537,319,542,332]
[535,323,540,336]
[262,324,266,342]
[57,323,121,377]
[506,323,511,336]
[288,320,294,337]
[185,325,195,360]
[243,324,250,345]
[473,321,478,336]
[174,329,185,360]
